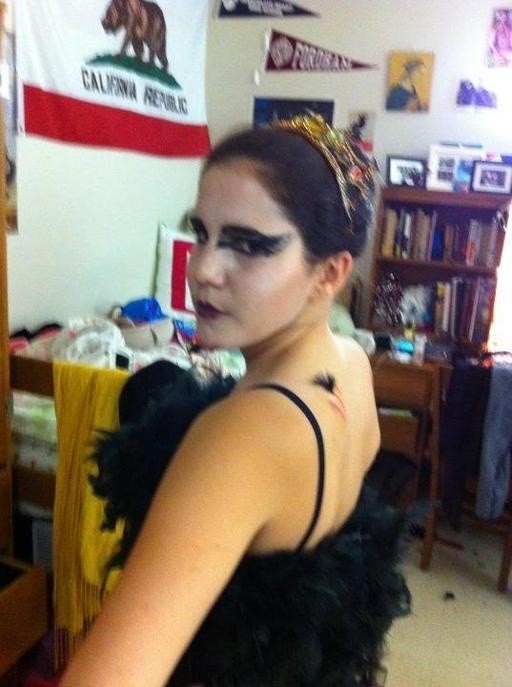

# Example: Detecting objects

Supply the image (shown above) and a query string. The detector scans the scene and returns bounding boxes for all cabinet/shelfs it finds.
[360,186,512,368]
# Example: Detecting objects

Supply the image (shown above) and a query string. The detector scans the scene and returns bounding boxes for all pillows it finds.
[154,223,197,322]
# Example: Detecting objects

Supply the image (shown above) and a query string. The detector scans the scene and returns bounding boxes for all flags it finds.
[12,0,214,159]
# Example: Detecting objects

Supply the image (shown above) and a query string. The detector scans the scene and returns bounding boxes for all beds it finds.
[11,316,360,564]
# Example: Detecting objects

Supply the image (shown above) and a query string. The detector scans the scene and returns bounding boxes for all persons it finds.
[385,57,428,112]
[58,112,414,686]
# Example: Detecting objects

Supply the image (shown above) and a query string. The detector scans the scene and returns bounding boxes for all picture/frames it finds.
[385,143,512,192]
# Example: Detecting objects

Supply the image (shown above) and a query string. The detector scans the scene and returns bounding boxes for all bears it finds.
[101,0,169,72]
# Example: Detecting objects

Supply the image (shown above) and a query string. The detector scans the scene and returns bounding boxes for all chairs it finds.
[368,354,511,595]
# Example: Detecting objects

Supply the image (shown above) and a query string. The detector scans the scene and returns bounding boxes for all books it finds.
[372,199,505,345]
